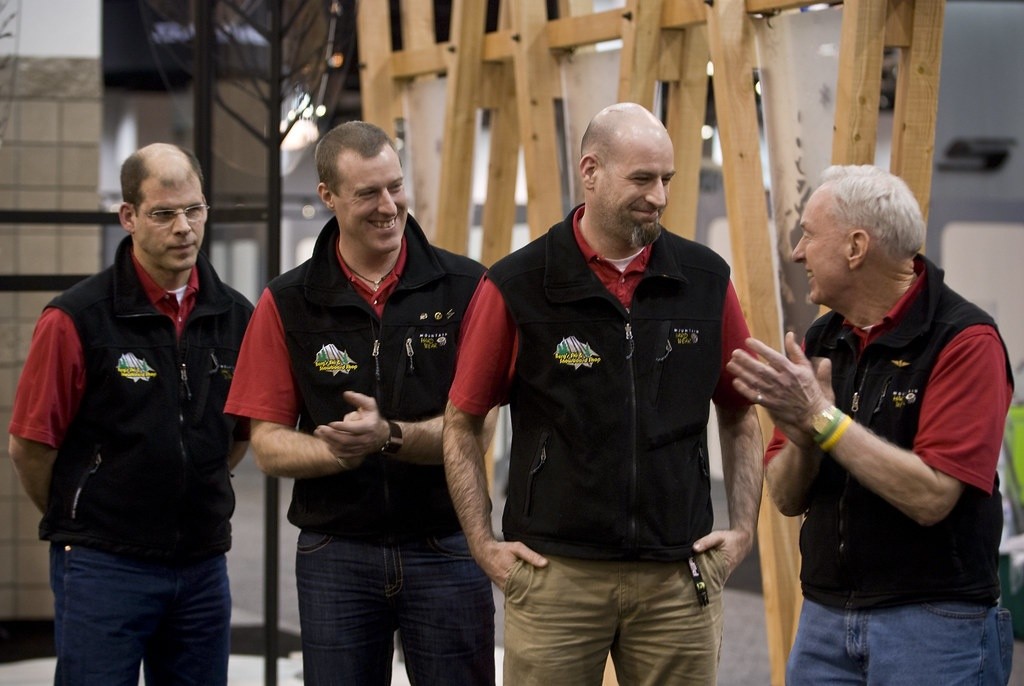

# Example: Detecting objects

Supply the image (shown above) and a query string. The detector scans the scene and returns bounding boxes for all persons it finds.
[726,165,1015,686]
[223,120,519,686]
[442,102,764,686]
[7,142,254,686]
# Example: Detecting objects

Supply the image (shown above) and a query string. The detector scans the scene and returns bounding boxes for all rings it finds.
[755,394,763,403]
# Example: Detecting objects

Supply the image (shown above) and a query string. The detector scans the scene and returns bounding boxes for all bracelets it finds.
[337,458,349,470]
[811,408,851,452]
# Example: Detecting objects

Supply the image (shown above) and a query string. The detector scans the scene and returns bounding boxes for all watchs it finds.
[806,406,840,433]
[380,418,404,456]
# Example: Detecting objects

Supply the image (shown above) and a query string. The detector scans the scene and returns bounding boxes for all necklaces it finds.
[339,251,395,292]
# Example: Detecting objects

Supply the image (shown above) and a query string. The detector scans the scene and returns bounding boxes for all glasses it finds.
[132,195,210,227]
